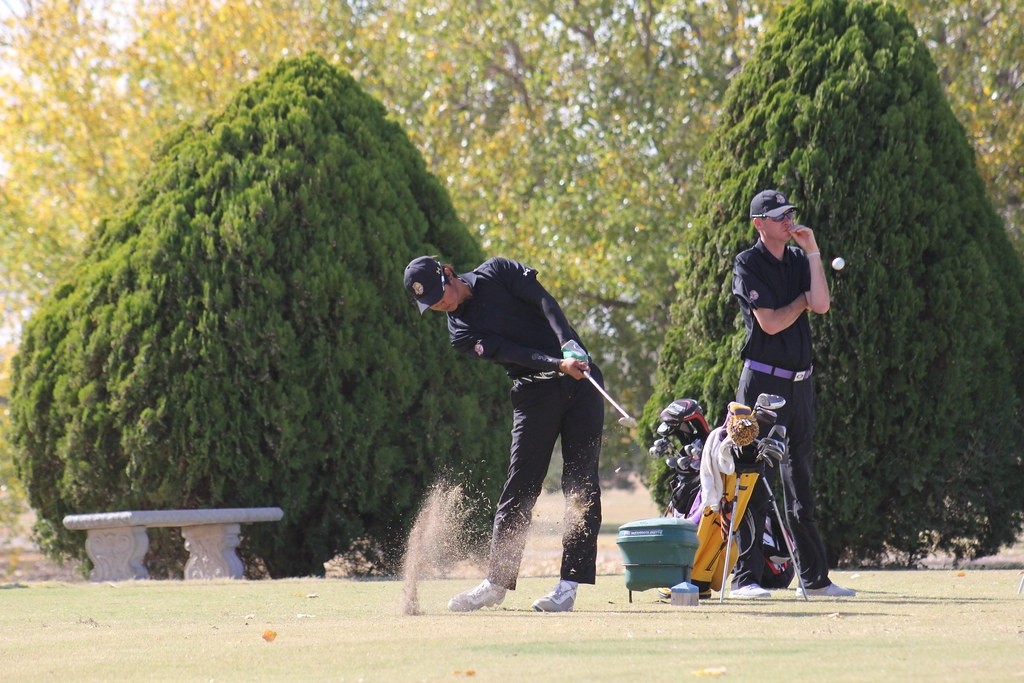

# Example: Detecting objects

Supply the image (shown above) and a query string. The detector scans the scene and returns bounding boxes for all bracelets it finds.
[807,252,820,258]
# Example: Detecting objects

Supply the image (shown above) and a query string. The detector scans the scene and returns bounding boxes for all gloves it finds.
[561,340,589,375]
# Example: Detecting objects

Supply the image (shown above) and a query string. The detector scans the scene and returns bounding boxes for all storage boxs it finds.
[614,517,698,590]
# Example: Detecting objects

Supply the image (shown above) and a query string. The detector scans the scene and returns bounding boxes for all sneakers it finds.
[532,582,576,613]
[728,582,771,599]
[448,579,506,612]
[796,582,856,598]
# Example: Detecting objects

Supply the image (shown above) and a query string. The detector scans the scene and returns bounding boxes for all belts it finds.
[744,359,813,382]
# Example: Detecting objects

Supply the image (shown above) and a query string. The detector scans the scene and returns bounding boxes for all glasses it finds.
[752,211,793,223]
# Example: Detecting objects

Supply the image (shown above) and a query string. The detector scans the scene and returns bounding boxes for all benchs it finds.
[62,506,284,581]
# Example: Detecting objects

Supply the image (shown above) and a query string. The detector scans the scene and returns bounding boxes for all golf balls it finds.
[832,257,845,270]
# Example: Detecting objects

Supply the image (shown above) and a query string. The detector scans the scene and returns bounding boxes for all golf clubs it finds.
[752,392,789,469]
[648,437,703,471]
[580,367,639,429]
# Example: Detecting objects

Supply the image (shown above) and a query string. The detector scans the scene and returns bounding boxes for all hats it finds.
[404,255,445,315]
[749,189,799,220]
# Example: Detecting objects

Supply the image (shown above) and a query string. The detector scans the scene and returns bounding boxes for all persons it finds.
[729,190,855,597]
[404,255,605,612]
